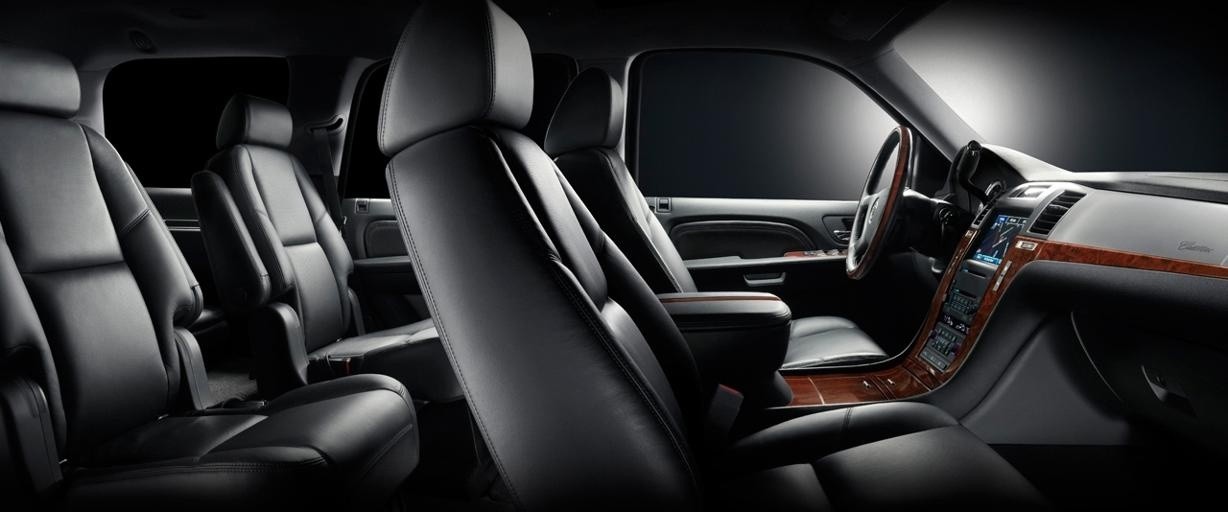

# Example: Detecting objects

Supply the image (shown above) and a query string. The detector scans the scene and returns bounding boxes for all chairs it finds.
[537,68,894,369]
[371,1,1073,511]
[187,86,468,403]
[1,26,428,509]
